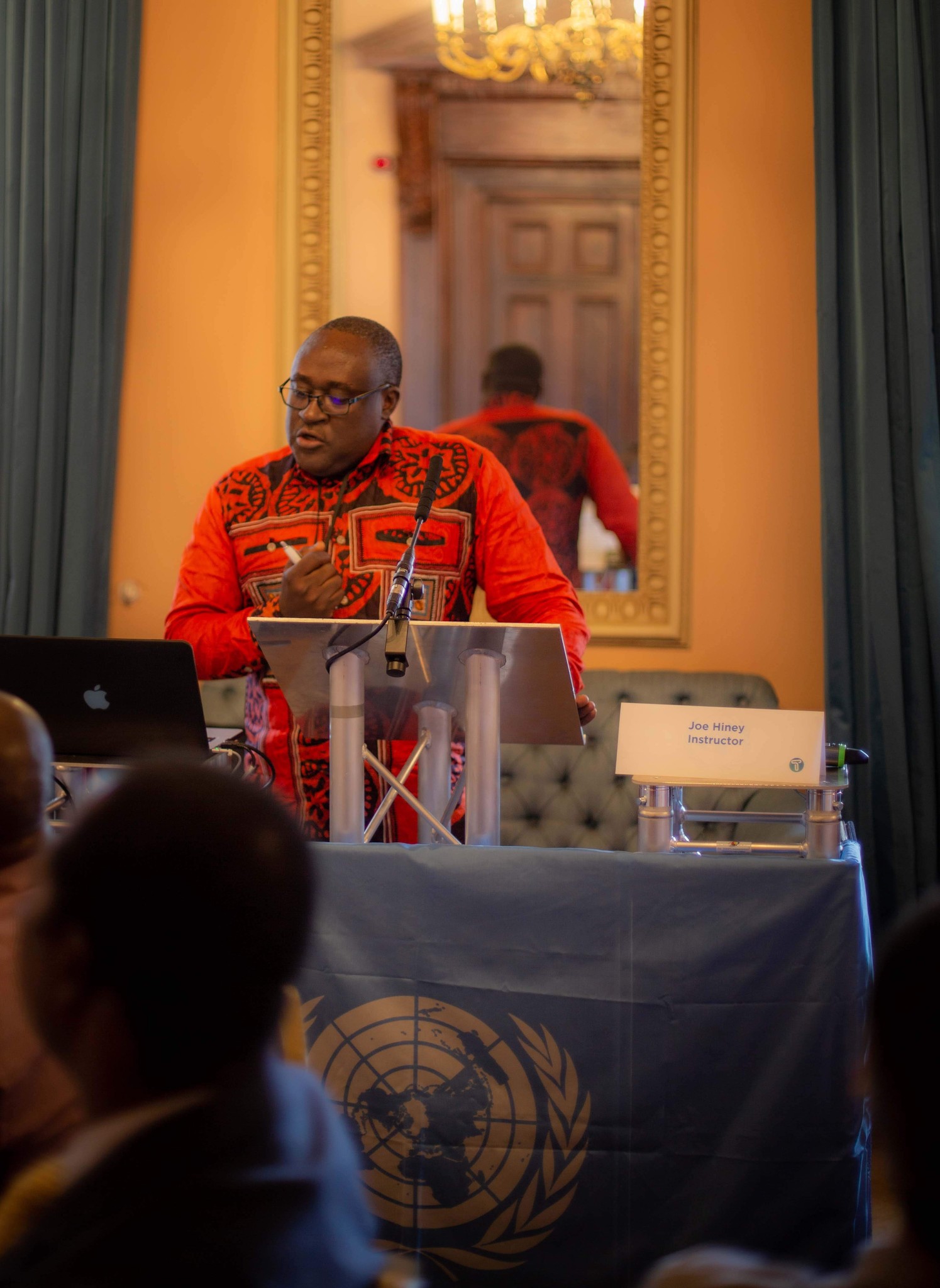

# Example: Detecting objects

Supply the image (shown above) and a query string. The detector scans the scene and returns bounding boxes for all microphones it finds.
[383,455,443,616]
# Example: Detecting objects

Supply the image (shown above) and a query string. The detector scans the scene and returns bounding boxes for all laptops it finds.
[0,633,243,768]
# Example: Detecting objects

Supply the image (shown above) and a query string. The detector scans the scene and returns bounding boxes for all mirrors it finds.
[284,2,690,641]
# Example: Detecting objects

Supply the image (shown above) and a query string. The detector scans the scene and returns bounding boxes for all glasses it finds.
[278,377,391,416]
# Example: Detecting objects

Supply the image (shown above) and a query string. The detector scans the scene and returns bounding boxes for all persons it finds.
[430,342,638,591]
[0,692,377,1288]
[163,316,596,845]
[647,885,940,1288]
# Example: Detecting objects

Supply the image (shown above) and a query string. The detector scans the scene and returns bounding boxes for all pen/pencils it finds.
[280,541,303,567]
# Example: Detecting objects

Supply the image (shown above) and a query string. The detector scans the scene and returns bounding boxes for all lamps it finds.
[434,3,646,104]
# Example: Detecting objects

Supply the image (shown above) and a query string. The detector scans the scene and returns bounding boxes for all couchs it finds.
[195,669,814,853]
